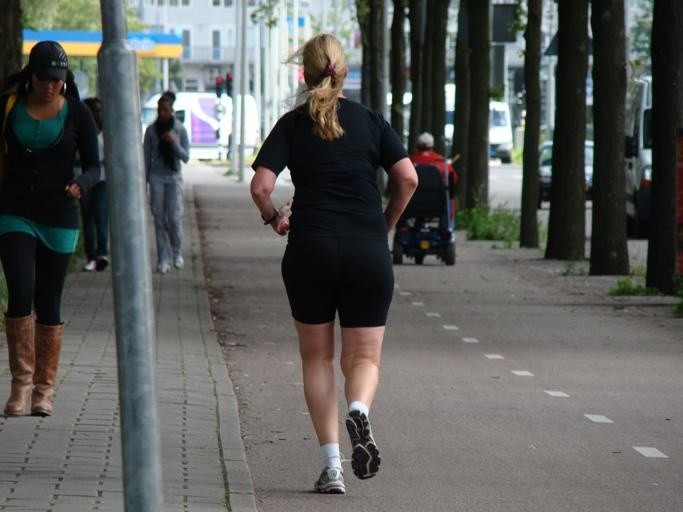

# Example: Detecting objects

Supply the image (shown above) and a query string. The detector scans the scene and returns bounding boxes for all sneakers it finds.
[83,255,109,273]
[312,465,348,494]
[343,409,382,481]
[155,252,188,275]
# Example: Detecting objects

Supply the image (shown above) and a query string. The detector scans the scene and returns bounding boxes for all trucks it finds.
[444,83,455,154]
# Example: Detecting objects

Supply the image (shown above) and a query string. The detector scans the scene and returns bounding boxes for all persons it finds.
[0,40,101,418]
[75,97,110,272]
[250,34,419,495]
[143,91,190,273]
[411,132,457,239]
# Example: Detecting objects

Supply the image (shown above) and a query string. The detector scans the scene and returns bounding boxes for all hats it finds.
[415,131,437,149]
[30,39,69,83]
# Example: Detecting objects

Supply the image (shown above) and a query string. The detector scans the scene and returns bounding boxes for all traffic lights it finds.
[225,72,232,97]
[215,77,222,96]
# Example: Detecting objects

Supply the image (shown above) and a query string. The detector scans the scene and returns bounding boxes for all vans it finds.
[488,100,513,163]
[140,89,257,148]
[624,76,652,233]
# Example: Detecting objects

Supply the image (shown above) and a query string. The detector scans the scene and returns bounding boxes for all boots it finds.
[31,321,64,416]
[4,312,34,418]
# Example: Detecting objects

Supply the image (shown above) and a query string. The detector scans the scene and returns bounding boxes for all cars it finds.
[537,140,594,209]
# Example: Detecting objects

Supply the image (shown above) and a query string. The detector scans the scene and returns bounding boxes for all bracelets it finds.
[261,207,278,225]
